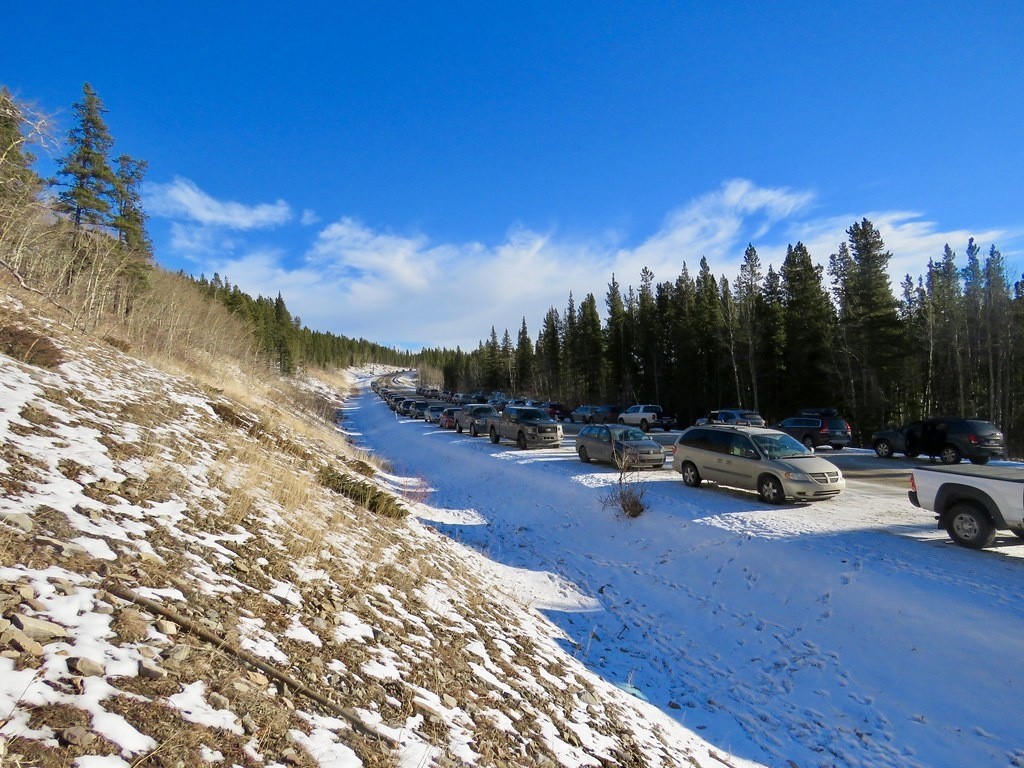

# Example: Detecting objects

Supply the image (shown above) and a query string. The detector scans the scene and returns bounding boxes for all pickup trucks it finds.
[618,405,677,432]
[486,406,564,449]
[908,463,1024,549]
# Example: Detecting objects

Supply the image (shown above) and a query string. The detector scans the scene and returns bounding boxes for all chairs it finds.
[622,431,631,441]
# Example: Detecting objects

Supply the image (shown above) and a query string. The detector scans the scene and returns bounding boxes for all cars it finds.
[456,404,501,436]
[439,408,462,429]
[871,416,1004,464]
[487,399,506,411]
[525,401,543,407]
[697,409,767,428]
[570,406,599,424]
[409,401,430,418]
[673,422,845,505]
[505,400,525,408]
[540,402,567,421]
[371,381,406,414]
[589,405,626,424]
[574,424,666,470]
[400,399,415,416]
[423,406,446,424]
[769,417,851,453]
[416,387,487,404]
